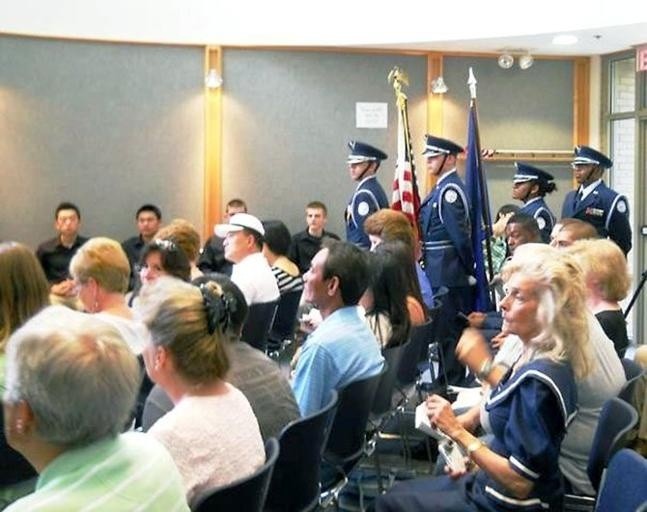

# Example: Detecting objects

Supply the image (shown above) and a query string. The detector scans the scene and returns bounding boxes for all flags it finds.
[388,67,425,272]
[464,65,496,310]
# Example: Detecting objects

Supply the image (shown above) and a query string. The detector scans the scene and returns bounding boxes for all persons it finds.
[1,131,647,511]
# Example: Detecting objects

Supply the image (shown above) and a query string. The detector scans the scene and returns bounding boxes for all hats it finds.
[346,141,388,164]
[420,134,463,158]
[213,213,266,236]
[513,162,554,183]
[569,145,613,169]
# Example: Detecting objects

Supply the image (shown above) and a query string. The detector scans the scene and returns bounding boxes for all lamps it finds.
[497,48,535,69]
[429,76,447,98]
[204,70,224,90]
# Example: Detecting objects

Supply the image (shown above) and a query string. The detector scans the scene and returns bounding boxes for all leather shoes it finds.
[416,379,444,391]
